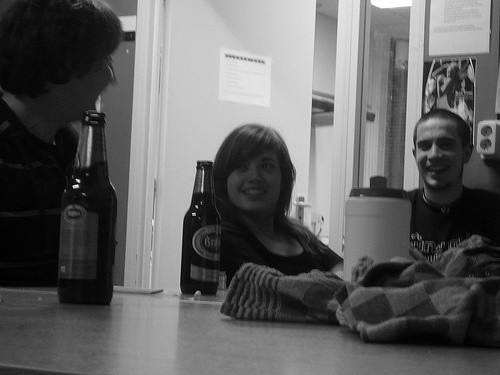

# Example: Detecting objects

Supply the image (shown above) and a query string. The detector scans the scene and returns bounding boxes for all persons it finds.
[211,122,344,299]
[425,58,476,125]
[405,108,500,283]
[0,0,124,288]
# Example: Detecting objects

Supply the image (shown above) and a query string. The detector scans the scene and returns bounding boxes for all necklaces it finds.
[422,187,466,214]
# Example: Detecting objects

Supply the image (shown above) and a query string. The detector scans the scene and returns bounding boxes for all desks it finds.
[0,285,500,375]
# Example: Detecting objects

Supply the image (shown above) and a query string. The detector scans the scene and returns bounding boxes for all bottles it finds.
[180,161,221,295]
[58,110,118,306]
